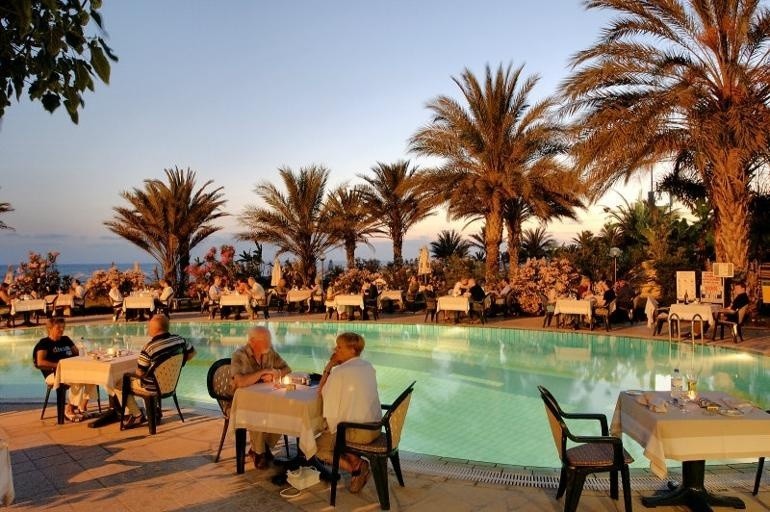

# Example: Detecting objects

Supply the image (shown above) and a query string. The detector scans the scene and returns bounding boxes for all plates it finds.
[625,390,645,396]
[716,405,749,417]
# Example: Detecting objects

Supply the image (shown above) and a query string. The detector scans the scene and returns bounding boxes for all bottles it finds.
[301,376,307,385]
[670,367,684,399]
[569,291,573,297]
[305,375,311,385]
[78,336,86,359]
[684,290,690,304]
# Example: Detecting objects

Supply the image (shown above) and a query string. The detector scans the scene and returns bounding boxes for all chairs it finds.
[206,358,287,462]
[592,298,616,332]
[538,385,636,511]
[0,290,89,329]
[710,303,749,343]
[120,352,184,434]
[647,294,678,338]
[537,291,556,328]
[330,380,416,510]
[32,344,101,420]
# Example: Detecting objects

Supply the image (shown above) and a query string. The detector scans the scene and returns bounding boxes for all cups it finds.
[272,369,282,385]
[685,366,704,401]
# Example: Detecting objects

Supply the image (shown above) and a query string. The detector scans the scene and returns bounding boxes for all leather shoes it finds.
[64,410,80,423]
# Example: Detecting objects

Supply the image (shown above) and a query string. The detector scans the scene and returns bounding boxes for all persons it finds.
[278,277,323,313]
[424,277,486,323]
[114,314,195,426]
[490,278,512,315]
[326,278,383,321]
[0,279,84,328]
[202,275,266,319]
[313,331,383,493]
[694,283,749,340]
[546,278,616,330]
[36,317,95,422]
[396,276,420,312]
[222,326,291,470]
[109,278,174,321]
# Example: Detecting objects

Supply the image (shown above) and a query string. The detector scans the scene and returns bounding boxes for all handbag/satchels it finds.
[287,465,321,491]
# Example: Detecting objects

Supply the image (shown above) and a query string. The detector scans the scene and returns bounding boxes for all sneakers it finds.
[123,409,147,428]
[350,461,372,493]
[248,443,273,470]
